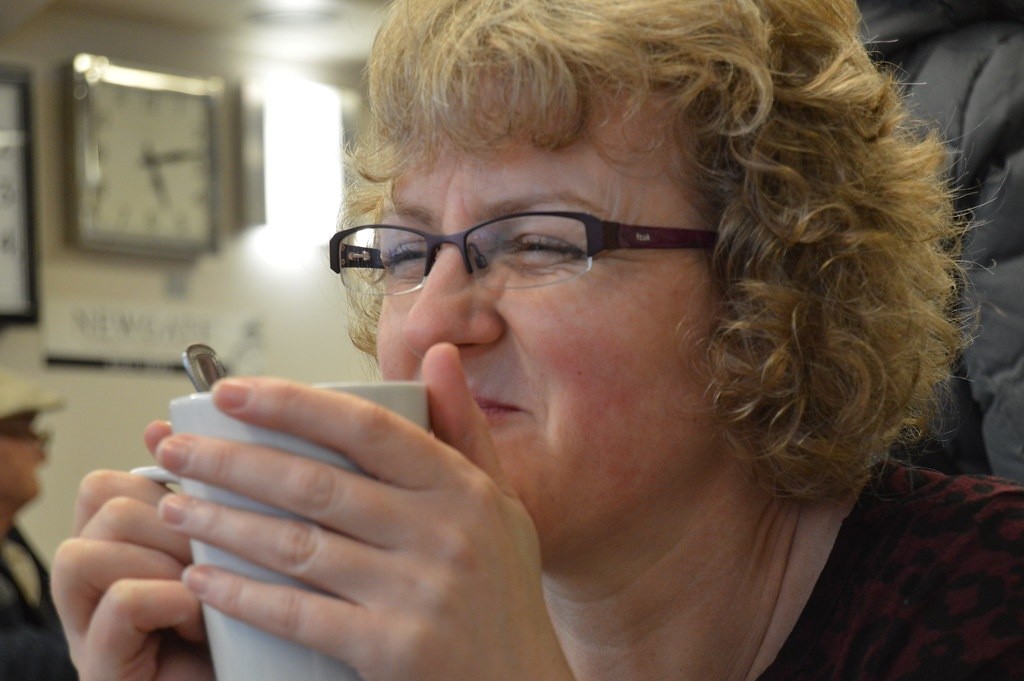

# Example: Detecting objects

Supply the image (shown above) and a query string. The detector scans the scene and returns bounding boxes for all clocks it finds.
[58,50,244,262]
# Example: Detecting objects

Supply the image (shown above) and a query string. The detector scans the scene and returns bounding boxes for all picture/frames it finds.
[1,63,42,326]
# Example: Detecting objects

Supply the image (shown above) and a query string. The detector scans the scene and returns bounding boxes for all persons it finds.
[1,364,81,681]
[45,0,1021,680]
[826,0,1022,490]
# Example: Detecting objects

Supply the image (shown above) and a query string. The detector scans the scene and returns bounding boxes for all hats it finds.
[0,366,68,417]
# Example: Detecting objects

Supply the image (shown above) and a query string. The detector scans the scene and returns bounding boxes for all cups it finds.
[128,381,430,679]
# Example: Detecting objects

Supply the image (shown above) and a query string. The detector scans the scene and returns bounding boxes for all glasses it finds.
[329,210,720,297]
[1,425,50,447]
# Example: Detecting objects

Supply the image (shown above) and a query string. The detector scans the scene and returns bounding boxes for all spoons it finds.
[181,342,226,393]
